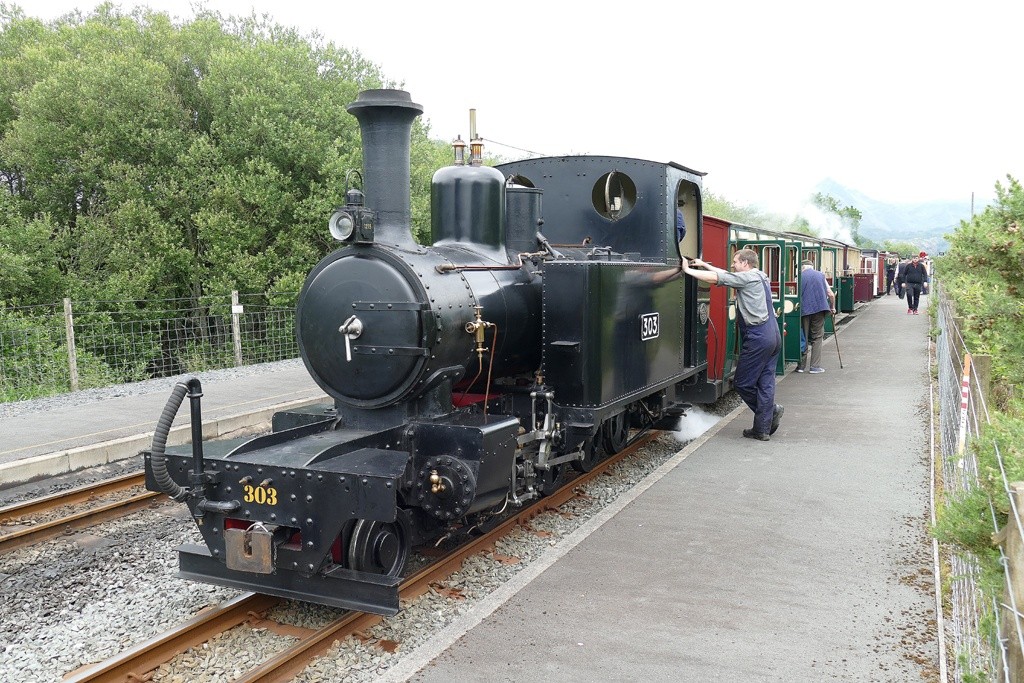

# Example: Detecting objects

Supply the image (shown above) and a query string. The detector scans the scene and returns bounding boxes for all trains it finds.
[144,88,898,617]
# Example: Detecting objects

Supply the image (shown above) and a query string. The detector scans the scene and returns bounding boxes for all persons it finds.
[793,260,837,374]
[610,263,681,394]
[885,254,929,314]
[682,249,784,440]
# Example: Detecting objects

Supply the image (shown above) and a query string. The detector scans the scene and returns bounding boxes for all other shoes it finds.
[913,309,919,315]
[907,308,913,314]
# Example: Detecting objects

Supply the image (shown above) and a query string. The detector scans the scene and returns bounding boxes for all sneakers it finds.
[796,366,805,373]
[808,366,825,373]
[743,428,769,441]
[769,404,784,436]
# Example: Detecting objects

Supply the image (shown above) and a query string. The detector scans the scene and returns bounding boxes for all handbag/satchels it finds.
[800,327,806,352]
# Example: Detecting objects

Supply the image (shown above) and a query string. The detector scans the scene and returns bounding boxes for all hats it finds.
[795,260,812,267]
